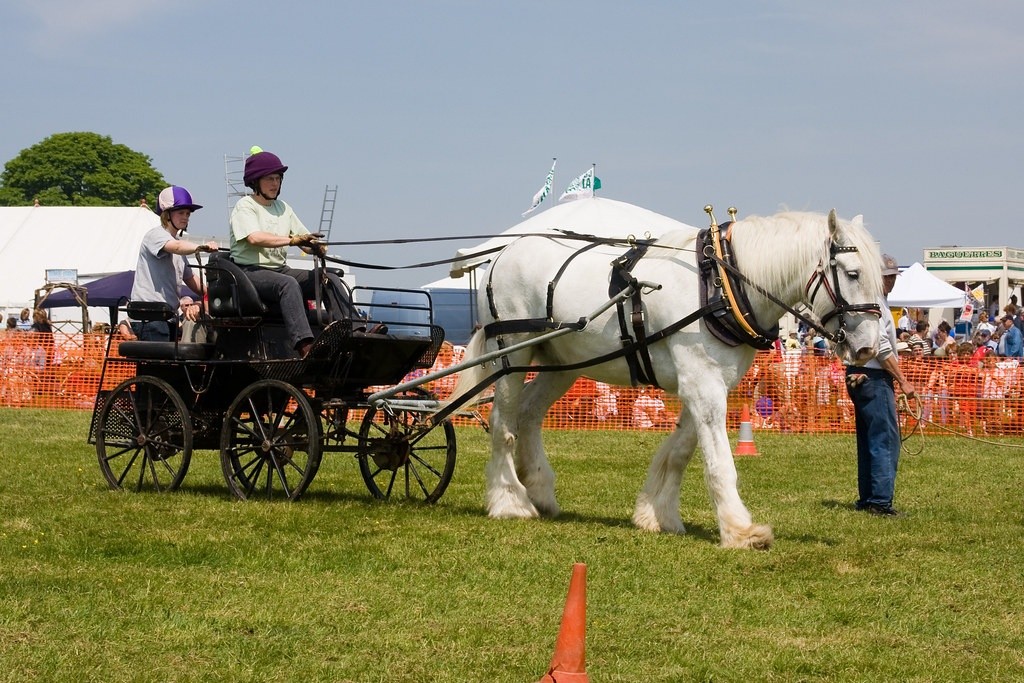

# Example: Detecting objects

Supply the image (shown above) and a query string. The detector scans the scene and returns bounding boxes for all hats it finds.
[1000,315,1013,321]
[789,330,797,336]
[978,329,991,335]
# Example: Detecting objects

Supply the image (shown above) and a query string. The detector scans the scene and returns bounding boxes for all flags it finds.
[521,160,594,217]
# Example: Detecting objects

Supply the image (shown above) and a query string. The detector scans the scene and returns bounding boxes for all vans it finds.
[364,286,481,349]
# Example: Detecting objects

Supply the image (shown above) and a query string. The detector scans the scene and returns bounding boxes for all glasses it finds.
[976,332,989,338]
[181,303,193,307]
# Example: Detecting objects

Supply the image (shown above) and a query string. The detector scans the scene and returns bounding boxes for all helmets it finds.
[243,151,288,182]
[156,185,203,213]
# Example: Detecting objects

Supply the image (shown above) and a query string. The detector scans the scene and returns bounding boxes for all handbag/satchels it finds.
[972,345,994,361]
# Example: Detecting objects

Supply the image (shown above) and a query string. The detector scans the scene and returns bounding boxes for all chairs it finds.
[205,253,344,328]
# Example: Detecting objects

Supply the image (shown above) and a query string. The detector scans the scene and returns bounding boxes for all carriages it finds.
[87,200,886,550]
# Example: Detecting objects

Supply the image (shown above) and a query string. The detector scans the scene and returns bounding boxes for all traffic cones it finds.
[537,563,590,683]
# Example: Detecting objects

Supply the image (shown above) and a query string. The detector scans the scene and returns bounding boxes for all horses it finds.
[423,197,885,552]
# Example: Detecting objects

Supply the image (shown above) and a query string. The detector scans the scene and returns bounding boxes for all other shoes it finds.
[368,323,388,335]
[854,500,898,516]
[297,342,313,358]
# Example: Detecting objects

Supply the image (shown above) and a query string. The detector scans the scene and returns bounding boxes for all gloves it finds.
[288,232,325,246]
[301,239,329,255]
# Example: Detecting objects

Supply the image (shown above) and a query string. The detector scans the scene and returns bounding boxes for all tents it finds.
[887,261,974,345]
[450,197,691,344]
[35,270,203,334]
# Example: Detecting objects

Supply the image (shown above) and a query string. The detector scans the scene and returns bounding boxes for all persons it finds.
[126,186,219,448]
[844,253,916,517]
[727,294,1024,435]
[348,340,681,432]
[0,294,219,410]
[227,146,388,360]
